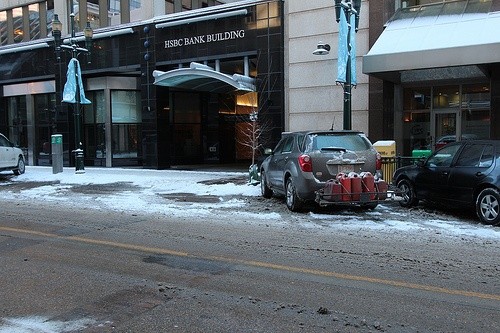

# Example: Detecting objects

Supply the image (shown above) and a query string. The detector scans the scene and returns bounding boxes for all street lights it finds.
[50,12,94,174]
[332,0,360,129]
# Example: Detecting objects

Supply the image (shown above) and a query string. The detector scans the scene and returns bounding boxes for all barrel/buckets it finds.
[323,179,342,202]
[360,171,375,200]
[335,172,351,201]
[348,171,361,200]
[374,177,388,200]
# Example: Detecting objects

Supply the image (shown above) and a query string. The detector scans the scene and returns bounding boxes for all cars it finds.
[436,135,479,148]
[393,141,499,225]
[0,133,25,177]
[259,130,387,211]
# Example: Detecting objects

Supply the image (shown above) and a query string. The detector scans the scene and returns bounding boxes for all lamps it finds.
[312,40,331,56]
